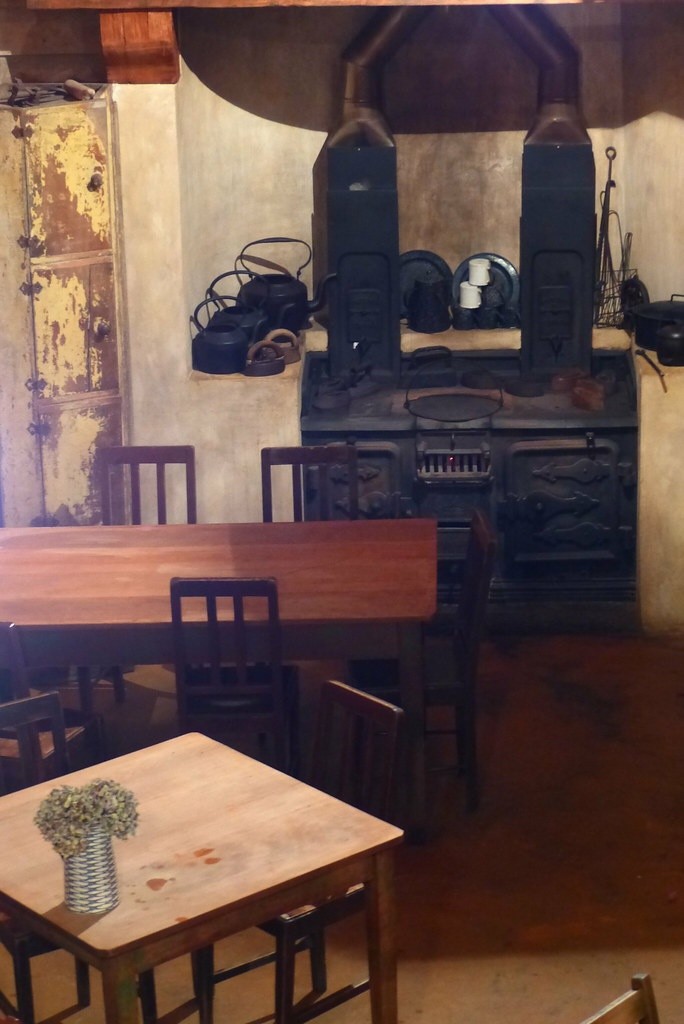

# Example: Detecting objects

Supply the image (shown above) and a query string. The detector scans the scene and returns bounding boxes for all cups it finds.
[454,258,521,330]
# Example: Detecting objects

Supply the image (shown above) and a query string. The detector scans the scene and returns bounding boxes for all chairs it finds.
[170,577,300,778]
[101,445,197,524]
[1,622,85,797]
[199,679,404,1024]
[261,446,358,523]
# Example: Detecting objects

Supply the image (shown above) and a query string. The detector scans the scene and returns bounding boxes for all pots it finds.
[629,294,684,351]
[657,319,684,366]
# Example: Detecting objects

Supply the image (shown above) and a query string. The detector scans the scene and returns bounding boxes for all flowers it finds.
[34,778,140,861]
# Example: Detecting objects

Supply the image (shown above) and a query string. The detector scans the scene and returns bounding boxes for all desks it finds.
[4,731,404,1024]
[0,519,437,845]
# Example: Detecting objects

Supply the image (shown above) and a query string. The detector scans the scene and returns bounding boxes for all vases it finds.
[63,820,120,916]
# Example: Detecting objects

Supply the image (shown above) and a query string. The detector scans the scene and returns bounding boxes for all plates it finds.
[399,250,453,314]
[454,253,520,310]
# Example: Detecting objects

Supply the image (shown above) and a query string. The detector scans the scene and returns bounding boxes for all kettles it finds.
[192,296,266,374]
[209,270,295,343]
[237,236,337,330]
[404,272,453,333]
